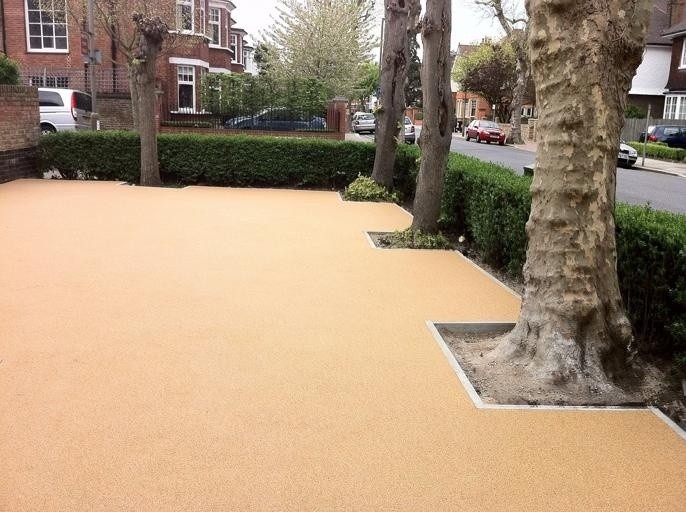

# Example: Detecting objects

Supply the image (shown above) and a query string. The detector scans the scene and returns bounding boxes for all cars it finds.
[616,139,638,170]
[224,106,327,131]
[465,119,506,146]
[404,117,416,143]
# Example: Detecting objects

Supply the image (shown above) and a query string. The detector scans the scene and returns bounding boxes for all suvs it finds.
[640,125,686,149]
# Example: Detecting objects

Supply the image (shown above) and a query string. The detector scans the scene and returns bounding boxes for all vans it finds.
[352,112,375,133]
[39,85,92,135]
